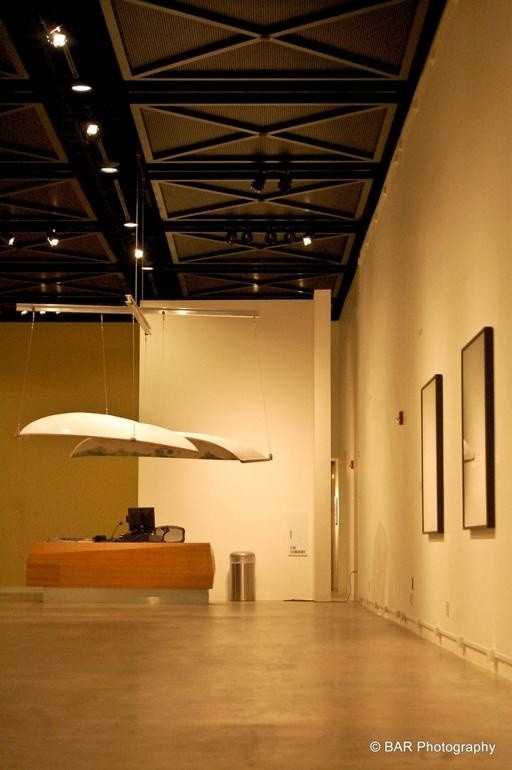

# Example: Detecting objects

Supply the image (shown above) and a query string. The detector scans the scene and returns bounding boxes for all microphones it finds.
[111,522,122,540]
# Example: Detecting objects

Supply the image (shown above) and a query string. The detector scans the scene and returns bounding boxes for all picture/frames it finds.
[422,328,493,532]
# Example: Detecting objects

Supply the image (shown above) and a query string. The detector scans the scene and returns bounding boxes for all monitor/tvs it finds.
[127,507,155,533]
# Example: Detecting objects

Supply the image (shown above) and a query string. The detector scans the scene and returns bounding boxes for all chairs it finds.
[151,526,185,542]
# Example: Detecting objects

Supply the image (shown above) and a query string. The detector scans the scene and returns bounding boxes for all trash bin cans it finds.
[231,552,256,601]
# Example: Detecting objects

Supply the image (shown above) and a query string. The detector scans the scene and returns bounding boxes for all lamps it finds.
[223,220,301,244]
[11,299,272,464]
[250,164,293,195]
[123,162,156,334]
